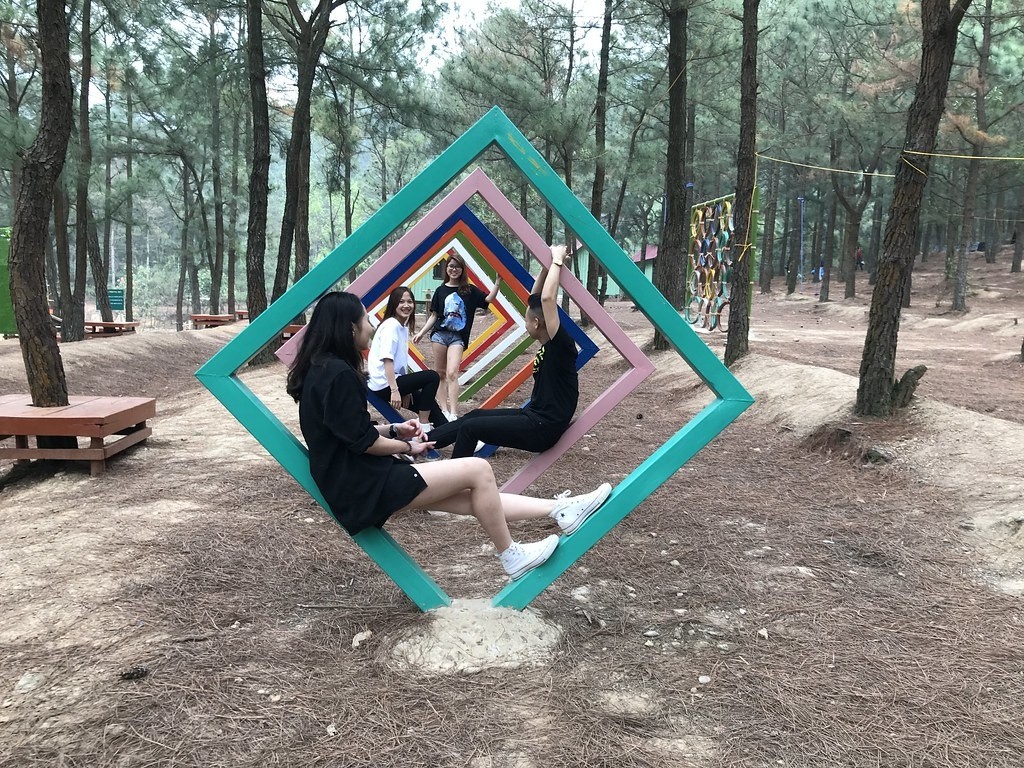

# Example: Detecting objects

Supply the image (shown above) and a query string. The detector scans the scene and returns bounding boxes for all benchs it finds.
[81,320,140,339]
[234,308,249,320]
[187,312,234,328]
[0,392,159,475]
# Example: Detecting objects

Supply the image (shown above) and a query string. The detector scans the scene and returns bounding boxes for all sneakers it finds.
[442,410,458,422]
[547,482,613,536]
[415,418,434,433]
[493,534,560,581]
[419,443,443,462]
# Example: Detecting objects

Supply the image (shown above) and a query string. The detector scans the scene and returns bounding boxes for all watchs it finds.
[389,423,398,438]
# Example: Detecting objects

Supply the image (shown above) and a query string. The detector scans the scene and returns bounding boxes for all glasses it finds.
[446,264,462,271]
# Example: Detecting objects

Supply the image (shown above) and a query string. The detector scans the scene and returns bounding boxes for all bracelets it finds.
[495,283,499,286]
[552,259,563,267]
[391,387,398,393]
[399,440,412,454]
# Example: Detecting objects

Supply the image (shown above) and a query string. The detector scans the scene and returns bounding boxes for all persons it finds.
[397,245,580,462]
[286,291,612,581]
[410,254,503,422]
[366,286,486,453]
[855,246,864,272]
